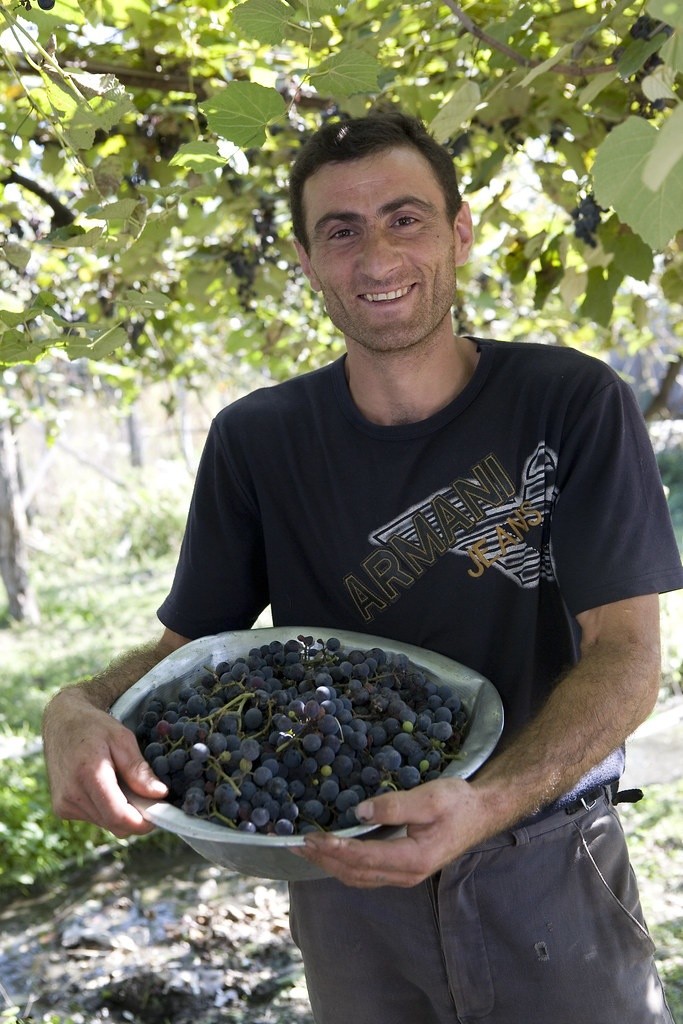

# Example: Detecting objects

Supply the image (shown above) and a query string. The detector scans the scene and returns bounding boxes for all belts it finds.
[503,782,606,836]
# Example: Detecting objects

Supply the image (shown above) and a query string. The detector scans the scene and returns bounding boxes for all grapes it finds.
[139,10,678,318]
[135,633,468,834]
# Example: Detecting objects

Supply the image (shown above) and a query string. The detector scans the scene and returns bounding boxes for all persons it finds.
[42,113,683,1024]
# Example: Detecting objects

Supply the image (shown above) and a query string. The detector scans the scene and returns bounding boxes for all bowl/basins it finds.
[106,626,505,882]
